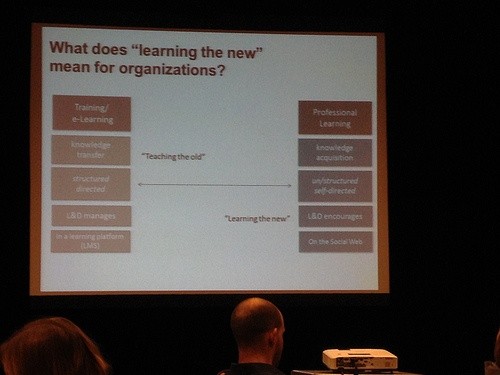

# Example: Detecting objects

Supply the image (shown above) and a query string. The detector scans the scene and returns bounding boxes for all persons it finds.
[0,317,108,375]
[217,298,284,374]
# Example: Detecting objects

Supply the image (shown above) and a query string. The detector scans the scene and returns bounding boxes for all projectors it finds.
[322,348,398,371]
